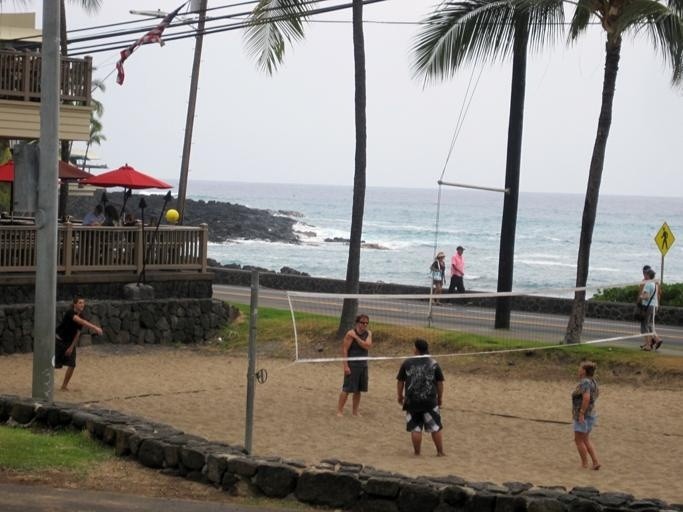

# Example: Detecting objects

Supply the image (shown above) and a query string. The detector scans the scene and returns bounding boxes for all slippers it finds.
[640,338,663,351]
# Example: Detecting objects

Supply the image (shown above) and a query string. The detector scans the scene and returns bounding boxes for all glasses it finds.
[358,321,368,324]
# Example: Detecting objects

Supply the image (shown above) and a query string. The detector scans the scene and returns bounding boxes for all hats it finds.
[457,247,466,250]
[437,251,445,257]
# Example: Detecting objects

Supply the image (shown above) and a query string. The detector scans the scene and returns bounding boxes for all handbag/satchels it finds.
[633,304,647,320]
[433,272,442,281]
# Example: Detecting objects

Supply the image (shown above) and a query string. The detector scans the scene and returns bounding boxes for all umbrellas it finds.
[0,156,174,193]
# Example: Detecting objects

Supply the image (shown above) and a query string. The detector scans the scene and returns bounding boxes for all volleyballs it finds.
[165,208,179,224]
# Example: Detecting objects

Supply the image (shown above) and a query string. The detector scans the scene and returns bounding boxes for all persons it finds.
[396,339,447,456]
[53,295,103,393]
[570,361,604,471]
[635,265,663,352]
[81,203,125,226]
[428,245,473,306]
[336,314,372,418]
